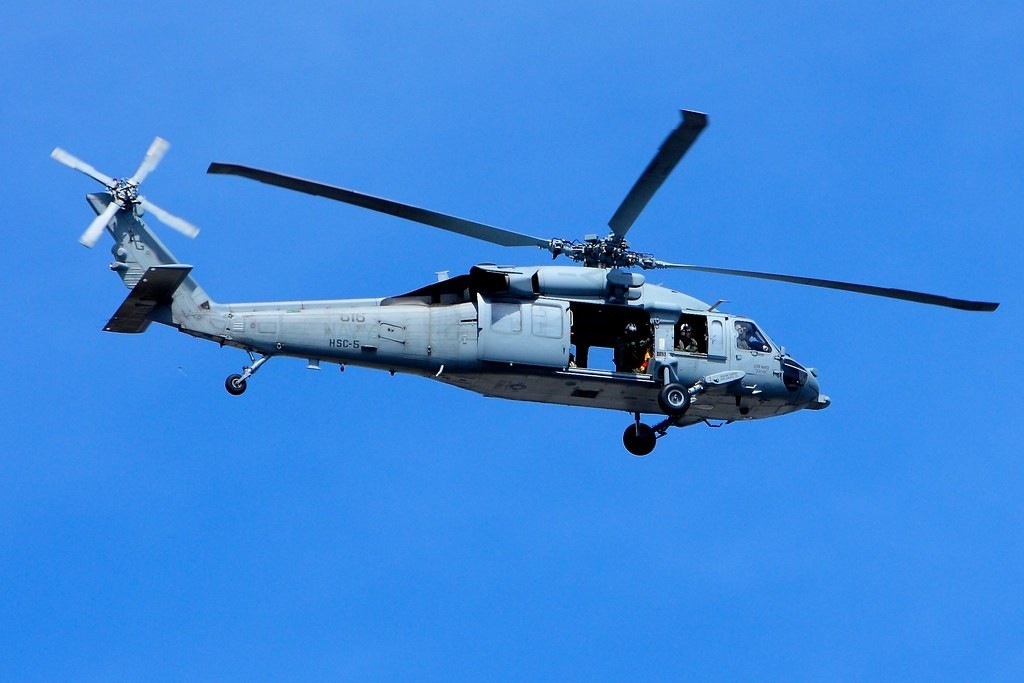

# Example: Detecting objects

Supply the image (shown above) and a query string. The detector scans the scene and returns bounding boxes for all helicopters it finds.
[48,106,1003,457]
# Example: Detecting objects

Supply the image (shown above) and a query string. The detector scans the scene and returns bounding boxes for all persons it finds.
[734,324,769,352]
[614,322,651,372]
[675,323,698,352]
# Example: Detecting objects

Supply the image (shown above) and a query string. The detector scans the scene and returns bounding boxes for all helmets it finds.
[735,324,746,338]
[624,322,637,335]
[680,323,692,335]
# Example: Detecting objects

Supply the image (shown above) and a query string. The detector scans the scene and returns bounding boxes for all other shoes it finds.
[632,368,642,375]
[569,361,577,368]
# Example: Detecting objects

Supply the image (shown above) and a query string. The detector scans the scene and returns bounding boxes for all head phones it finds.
[680,326,688,336]
[739,328,743,334]
